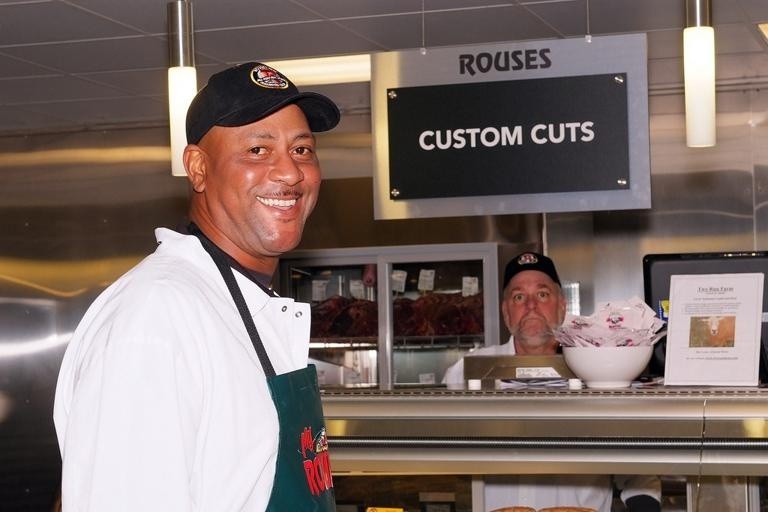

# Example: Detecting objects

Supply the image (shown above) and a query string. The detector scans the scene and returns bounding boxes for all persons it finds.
[52,65,339,511]
[441,250,661,511]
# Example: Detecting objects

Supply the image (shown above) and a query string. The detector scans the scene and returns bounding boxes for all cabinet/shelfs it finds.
[272,242,528,380]
[318,382,767,512]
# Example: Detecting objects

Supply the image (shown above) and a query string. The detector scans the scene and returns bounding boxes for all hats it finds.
[502,252,561,288]
[186,62,339,145]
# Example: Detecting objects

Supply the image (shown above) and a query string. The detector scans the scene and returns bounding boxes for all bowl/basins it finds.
[562,345,654,390]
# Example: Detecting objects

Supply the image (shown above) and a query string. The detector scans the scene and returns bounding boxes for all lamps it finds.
[162,2,205,181]
[678,0,720,152]
